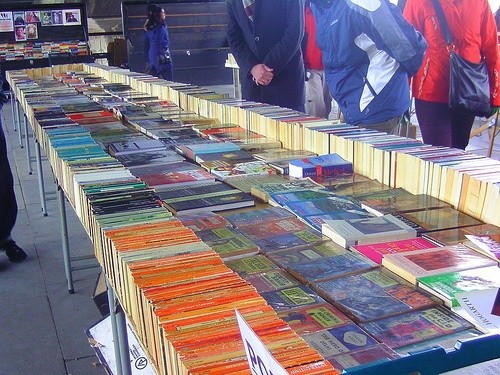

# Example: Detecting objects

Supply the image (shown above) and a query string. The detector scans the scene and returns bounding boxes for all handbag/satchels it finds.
[448,50,490,114]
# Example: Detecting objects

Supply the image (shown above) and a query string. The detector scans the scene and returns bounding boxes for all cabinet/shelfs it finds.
[121,0,233,85]
[1,2,94,95]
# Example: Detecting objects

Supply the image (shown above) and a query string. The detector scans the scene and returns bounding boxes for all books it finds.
[0,39,500,375]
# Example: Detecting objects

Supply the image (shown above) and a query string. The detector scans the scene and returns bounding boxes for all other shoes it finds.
[0,239,27,262]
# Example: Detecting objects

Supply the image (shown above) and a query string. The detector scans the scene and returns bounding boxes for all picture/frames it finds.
[62,9,82,26]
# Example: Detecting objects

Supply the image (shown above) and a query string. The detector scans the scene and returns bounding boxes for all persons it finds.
[226,0,305,113]
[14,13,24,25]
[301,0,500,150]
[142,3,174,82]
[16,28,26,39]
[41,13,52,25]
[0,73,27,262]
[67,15,78,22]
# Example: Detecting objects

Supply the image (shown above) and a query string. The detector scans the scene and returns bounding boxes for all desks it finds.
[0,63,500,375]
